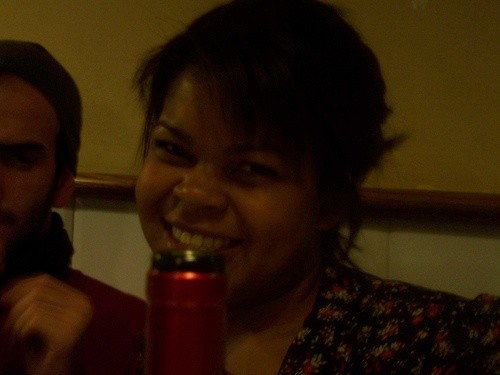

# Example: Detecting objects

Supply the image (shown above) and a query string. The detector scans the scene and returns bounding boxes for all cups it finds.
[148,249,225,375]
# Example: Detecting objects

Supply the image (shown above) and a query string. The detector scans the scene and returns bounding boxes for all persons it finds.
[130,0,500,375]
[0,39,151,375]
